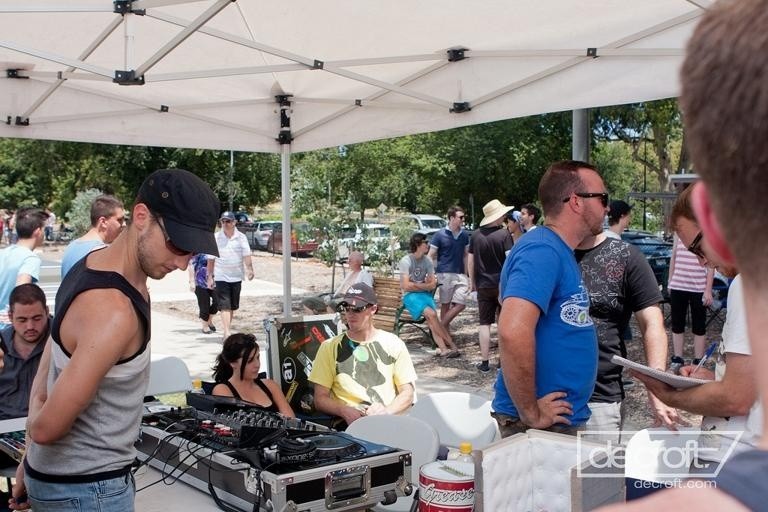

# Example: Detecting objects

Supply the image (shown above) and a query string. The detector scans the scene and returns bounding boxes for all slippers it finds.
[452,349,460,356]
[439,351,456,357]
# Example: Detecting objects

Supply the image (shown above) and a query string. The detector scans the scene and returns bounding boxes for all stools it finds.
[0,465,19,497]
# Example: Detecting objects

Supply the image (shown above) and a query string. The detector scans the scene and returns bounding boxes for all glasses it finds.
[345,304,368,313]
[419,240,429,245]
[152,213,201,256]
[563,192,608,207]
[453,216,465,220]
[225,334,257,356]
[687,229,707,260]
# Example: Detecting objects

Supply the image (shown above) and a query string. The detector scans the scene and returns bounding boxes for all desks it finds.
[129,461,416,512]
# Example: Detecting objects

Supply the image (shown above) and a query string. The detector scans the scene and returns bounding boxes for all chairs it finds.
[143,357,193,397]
[345,415,440,489]
[405,392,497,452]
[190,372,266,396]
[704,278,733,332]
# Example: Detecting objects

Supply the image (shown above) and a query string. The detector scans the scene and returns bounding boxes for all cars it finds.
[625,233,729,300]
[393,215,446,247]
[267,220,327,256]
[316,223,400,263]
[235,214,283,248]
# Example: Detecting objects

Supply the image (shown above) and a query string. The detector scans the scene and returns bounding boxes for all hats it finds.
[607,200,636,218]
[140,169,221,258]
[337,283,377,307]
[480,199,514,227]
[219,210,235,222]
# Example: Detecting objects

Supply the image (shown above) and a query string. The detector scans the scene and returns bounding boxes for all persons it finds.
[8,170,220,512]
[581,1,764,512]
[466,198,516,372]
[397,233,459,361]
[0,284,54,422]
[507,212,521,244]
[303,251,375,315]
[667,231,713,371]
[205,212,256,340]
[210,334,296,419]
[308,284,417,425]
[60,197,127,284]
[189,253,220,334]
[0,207,51,332]
[426,207,468,336]
[628,179,755,481]
[575,228,694,447]
[491,160,610,437]
[0,203,55,242]
[521,205,541,228]
[604,201,632,239]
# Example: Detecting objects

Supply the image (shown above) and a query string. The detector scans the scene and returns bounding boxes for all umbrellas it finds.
[1,1,715,317]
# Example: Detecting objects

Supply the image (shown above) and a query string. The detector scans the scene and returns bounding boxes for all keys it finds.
[14,494,29,505]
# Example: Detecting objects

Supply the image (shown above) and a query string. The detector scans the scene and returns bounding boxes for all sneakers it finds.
[666,361,684,375]
[476,363,489,373]
[693,359,706,369]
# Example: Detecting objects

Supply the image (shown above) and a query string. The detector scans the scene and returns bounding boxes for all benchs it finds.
[312,276,443,350]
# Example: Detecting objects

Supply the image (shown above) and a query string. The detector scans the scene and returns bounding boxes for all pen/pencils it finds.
[691,342,716,377]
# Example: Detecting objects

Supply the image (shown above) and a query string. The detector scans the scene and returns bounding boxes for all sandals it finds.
[208,323,216,331]
[202,328,211,334]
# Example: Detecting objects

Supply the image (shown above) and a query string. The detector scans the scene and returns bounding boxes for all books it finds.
[611,356,715,390]
